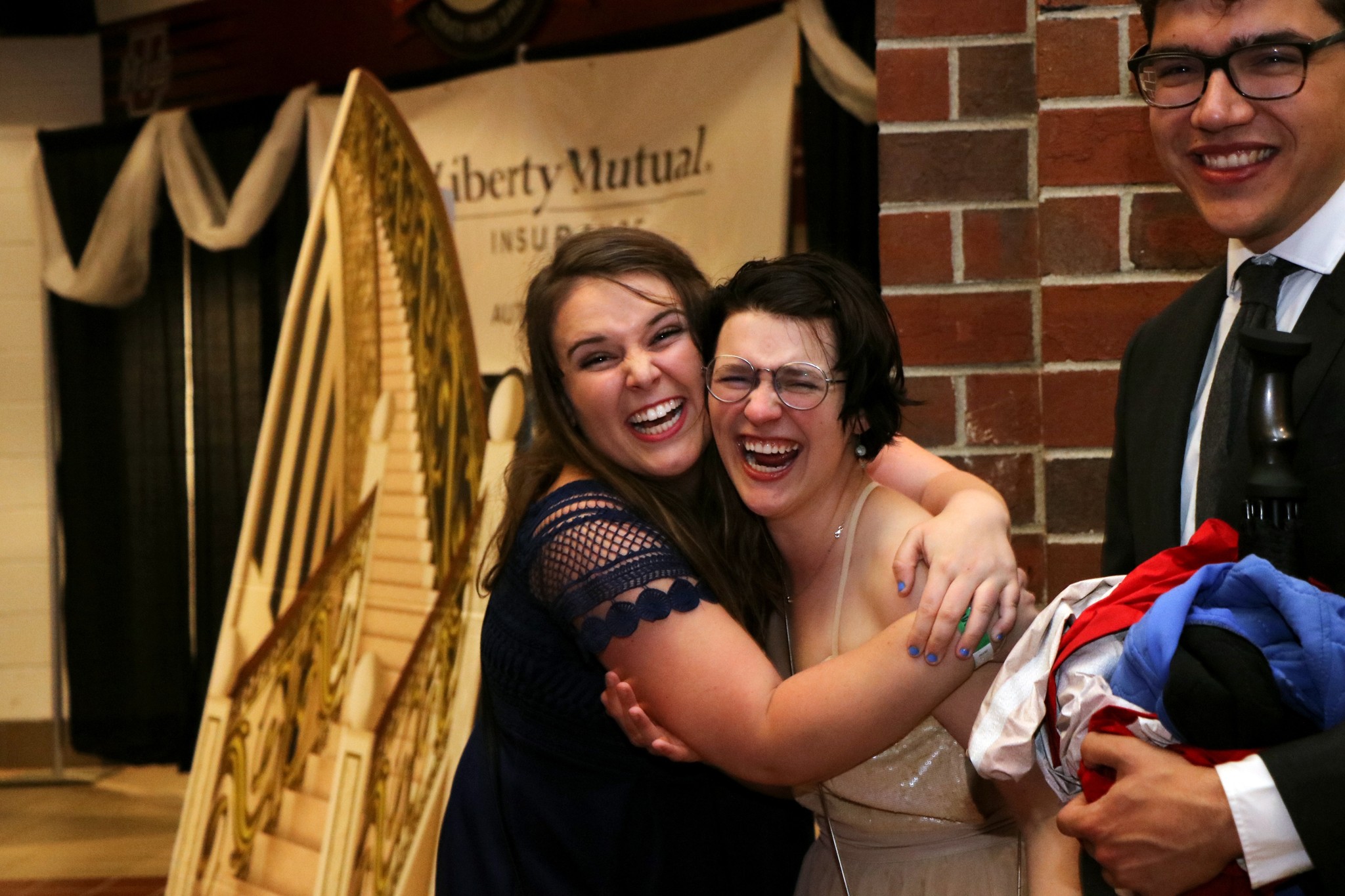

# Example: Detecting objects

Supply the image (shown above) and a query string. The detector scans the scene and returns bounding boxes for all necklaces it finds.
[762,461,861,603]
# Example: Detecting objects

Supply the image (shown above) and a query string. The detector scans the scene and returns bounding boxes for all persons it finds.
[434,230,1082,896]
[1056,0,1345,896]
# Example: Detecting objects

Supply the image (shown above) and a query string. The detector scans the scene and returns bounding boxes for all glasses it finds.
[700,354,849,409]
[1128,30,1345,108]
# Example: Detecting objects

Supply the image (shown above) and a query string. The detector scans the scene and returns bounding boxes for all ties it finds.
[1196,259,1302,528]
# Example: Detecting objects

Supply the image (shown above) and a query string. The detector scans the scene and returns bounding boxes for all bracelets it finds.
[957,605,996,669]
